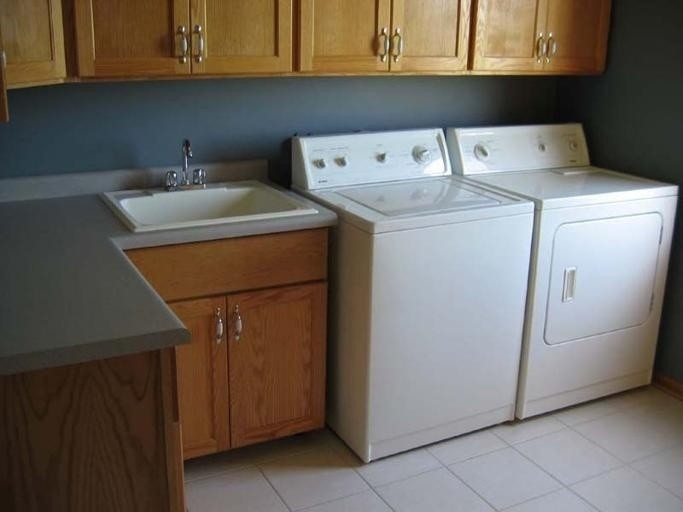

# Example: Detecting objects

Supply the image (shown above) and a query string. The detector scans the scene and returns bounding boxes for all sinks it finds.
[96,180,319,233]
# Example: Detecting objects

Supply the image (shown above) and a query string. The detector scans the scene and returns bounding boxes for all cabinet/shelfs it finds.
[296,0,469,74]
[73,1,294,81]
[0,0,66,90]
[168,280,328,462]
[469,0,612,72]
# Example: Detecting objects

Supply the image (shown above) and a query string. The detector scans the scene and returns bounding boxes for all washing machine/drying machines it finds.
[446,122,678,419]
[291,126,533,465]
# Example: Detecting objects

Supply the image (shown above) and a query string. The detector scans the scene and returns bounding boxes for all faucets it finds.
[164,141,206,188]
